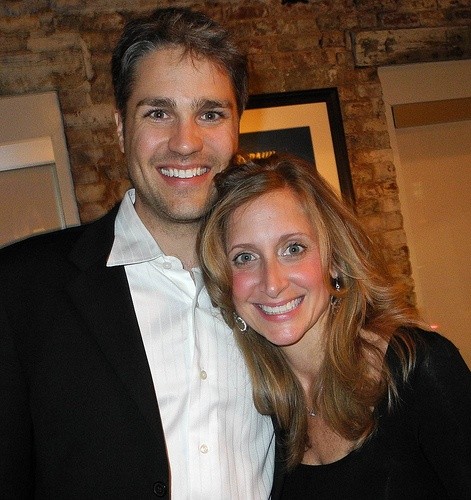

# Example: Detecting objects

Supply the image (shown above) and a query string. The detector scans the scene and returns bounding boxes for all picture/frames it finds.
[230,86,357,223]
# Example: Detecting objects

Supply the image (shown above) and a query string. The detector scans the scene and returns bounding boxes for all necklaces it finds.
[303,385,326,417]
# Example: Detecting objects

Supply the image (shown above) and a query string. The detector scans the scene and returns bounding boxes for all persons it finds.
[197,155,471,500]
[0,7,278,500]
